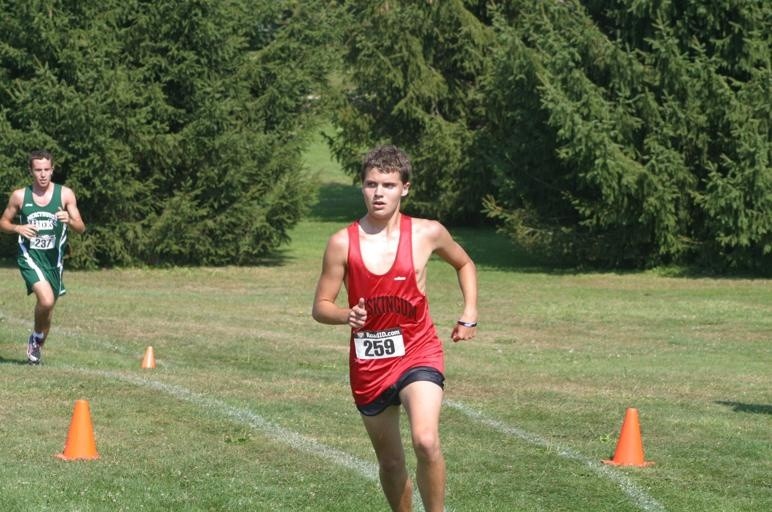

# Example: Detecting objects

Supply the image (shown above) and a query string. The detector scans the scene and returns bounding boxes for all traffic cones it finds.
[54,400,100,461]
[601,407,655,466]
[141,346,156,368]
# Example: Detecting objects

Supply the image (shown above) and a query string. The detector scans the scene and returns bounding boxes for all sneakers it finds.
[26,330,45,366]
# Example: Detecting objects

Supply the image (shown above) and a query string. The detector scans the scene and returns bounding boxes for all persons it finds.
[311,145,479,510]
[0,151,89,365]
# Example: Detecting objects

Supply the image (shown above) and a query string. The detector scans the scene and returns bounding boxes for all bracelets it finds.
[458,319,478,329]
